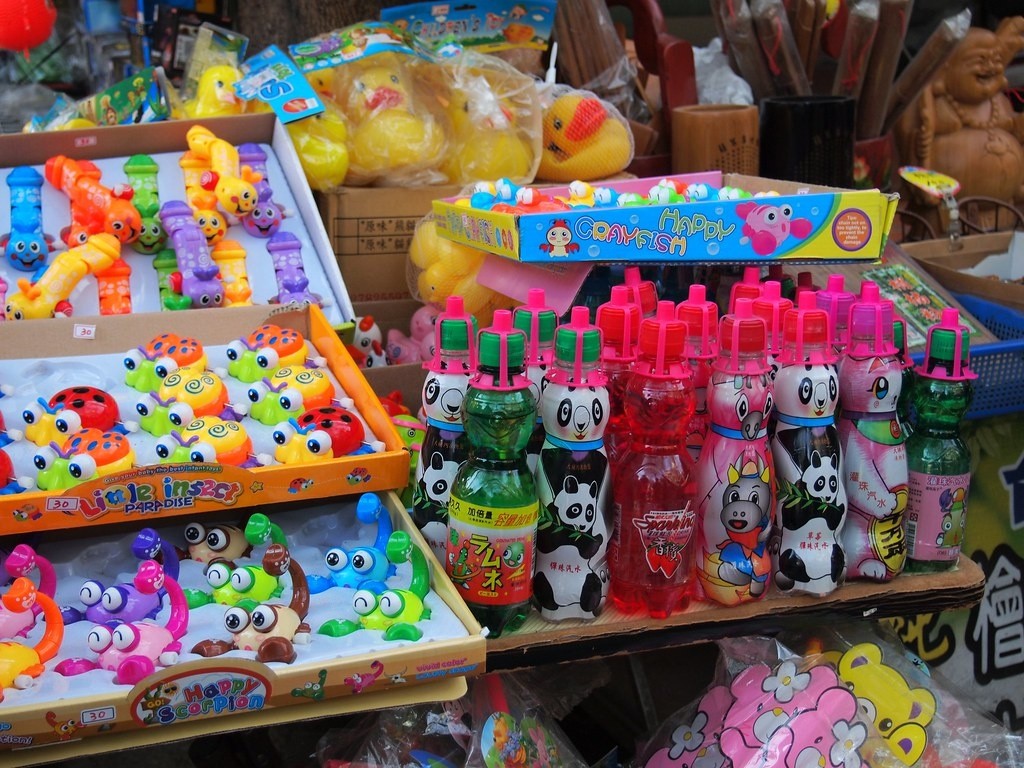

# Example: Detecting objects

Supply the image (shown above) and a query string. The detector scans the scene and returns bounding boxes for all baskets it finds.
[902,291,1024,419]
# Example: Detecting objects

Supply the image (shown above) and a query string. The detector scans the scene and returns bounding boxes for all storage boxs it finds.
[0,494,489,751]
[904,292,1024,423]
[897,228,1024,311]
[0,303,409,536]
[429,172,899,275]
[1,112,359,340]
[315,180,458,346]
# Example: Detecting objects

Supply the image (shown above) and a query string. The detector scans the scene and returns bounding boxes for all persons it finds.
[892,28,1024,238]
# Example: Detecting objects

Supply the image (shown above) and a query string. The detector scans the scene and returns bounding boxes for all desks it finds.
[490,552,986,755]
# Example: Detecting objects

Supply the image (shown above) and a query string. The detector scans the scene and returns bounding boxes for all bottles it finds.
[446,310,538,641]
[535,305,614,622]
[752,281,794,446]
[592,286,644,478]
[694,298,777,607]
[727,266,768,317]
[767,290,848,596]
[901,309,979,574]
[760,265,798,304]
[608,299,699,620]
[410,295,480,574]
[815,274,856,372]
[510,287,562,483]
[791,270,821,311]
[674,283,722,470]
[617,266,658,324]
[837,280,909,584]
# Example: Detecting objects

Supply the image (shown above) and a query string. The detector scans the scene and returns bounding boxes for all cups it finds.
[770,96,854,189]
[853,128,895,194]
[671,103,760,177]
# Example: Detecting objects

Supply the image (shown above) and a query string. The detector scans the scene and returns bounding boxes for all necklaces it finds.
[947,95,999,128]
